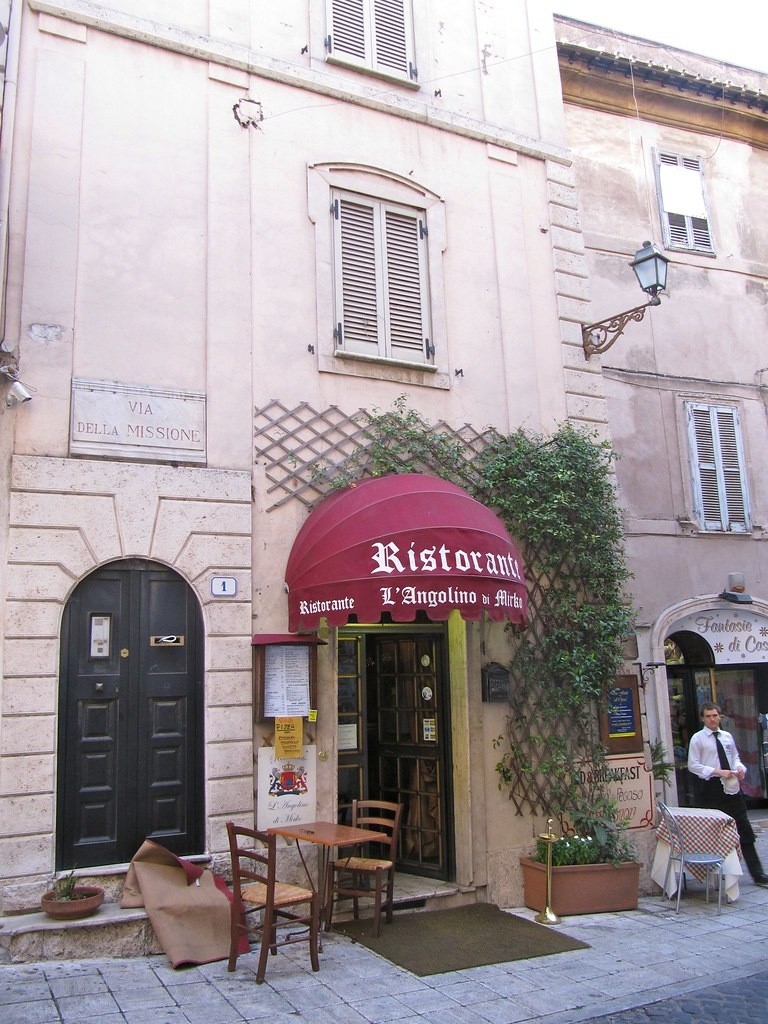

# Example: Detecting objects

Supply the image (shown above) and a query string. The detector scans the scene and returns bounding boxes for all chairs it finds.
[324,799,404,937]
[226,821,319,985]
[658,800,723,916]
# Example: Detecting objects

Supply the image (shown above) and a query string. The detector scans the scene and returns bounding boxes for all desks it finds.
[267,821,386,954]
[651,807,742,904]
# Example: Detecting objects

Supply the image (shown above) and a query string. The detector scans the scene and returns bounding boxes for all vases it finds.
[520,856,644,916]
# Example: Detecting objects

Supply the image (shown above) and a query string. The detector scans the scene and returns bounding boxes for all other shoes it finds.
[754,874,768,888]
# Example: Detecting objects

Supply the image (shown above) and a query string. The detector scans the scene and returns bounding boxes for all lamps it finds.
[580,240,672,362]
[719,592,753,605]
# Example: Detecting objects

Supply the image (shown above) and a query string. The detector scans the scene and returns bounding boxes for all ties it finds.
[713,732,730,770]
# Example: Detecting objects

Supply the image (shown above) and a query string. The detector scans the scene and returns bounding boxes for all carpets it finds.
[332,902,591,977]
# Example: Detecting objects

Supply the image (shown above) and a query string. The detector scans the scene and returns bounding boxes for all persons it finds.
[687,703,768,888]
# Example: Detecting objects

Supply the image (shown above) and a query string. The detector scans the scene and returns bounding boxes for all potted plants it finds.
[41,863,104,919]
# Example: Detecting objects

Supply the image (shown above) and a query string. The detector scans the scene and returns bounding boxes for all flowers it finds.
[529,799,638,869]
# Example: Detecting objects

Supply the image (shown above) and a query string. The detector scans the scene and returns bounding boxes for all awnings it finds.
[283,473,528,635]
